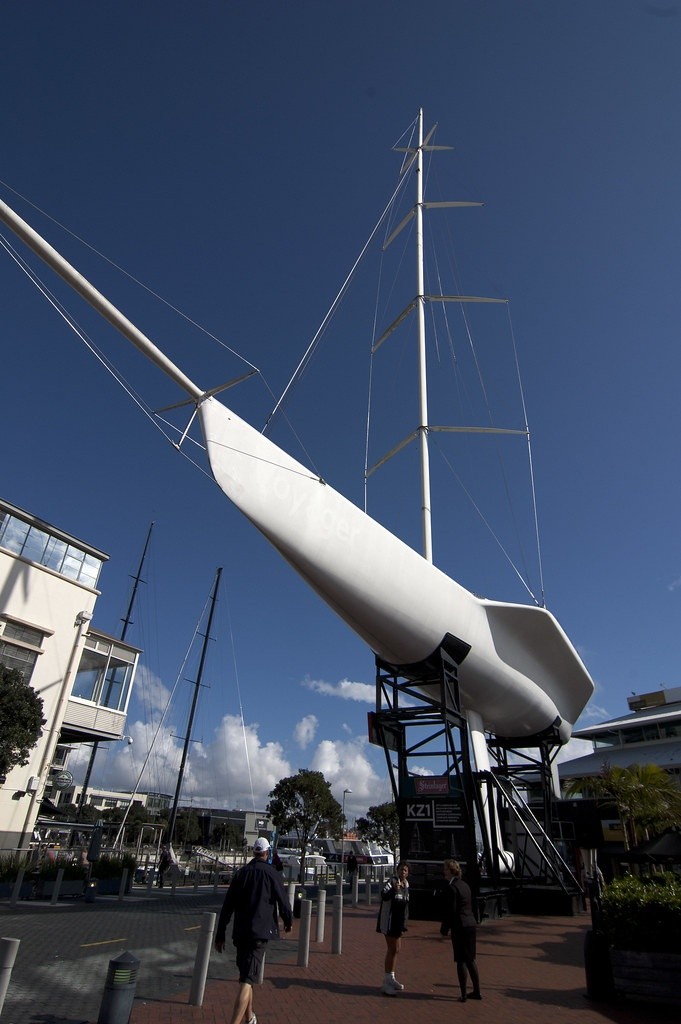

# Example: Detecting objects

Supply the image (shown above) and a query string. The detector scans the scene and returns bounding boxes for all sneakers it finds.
[247,1014,258,1023]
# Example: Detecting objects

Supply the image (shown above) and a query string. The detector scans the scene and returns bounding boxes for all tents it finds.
[619,831,681,876]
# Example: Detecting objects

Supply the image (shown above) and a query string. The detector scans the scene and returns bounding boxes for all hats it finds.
[253,837,272,854]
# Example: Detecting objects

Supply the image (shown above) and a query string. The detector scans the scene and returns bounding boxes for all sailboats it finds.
[2,106,597,745]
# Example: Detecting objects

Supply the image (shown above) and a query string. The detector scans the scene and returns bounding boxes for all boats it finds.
[276,854,339,882]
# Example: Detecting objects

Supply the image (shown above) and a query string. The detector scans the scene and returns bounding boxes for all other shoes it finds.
[459,991,482,1003]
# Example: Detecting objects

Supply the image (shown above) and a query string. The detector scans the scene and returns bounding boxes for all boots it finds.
[381,971,402,995]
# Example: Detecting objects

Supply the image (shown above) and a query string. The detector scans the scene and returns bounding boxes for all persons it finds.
[376,861,410,995]
[587,861,606,911]
[155,846,171,888]
[347,851,358,886]
[440,860,482,1002]
[215,837,293,1024]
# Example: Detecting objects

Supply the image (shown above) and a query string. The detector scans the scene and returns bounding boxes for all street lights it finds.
[342,787,352,880]
